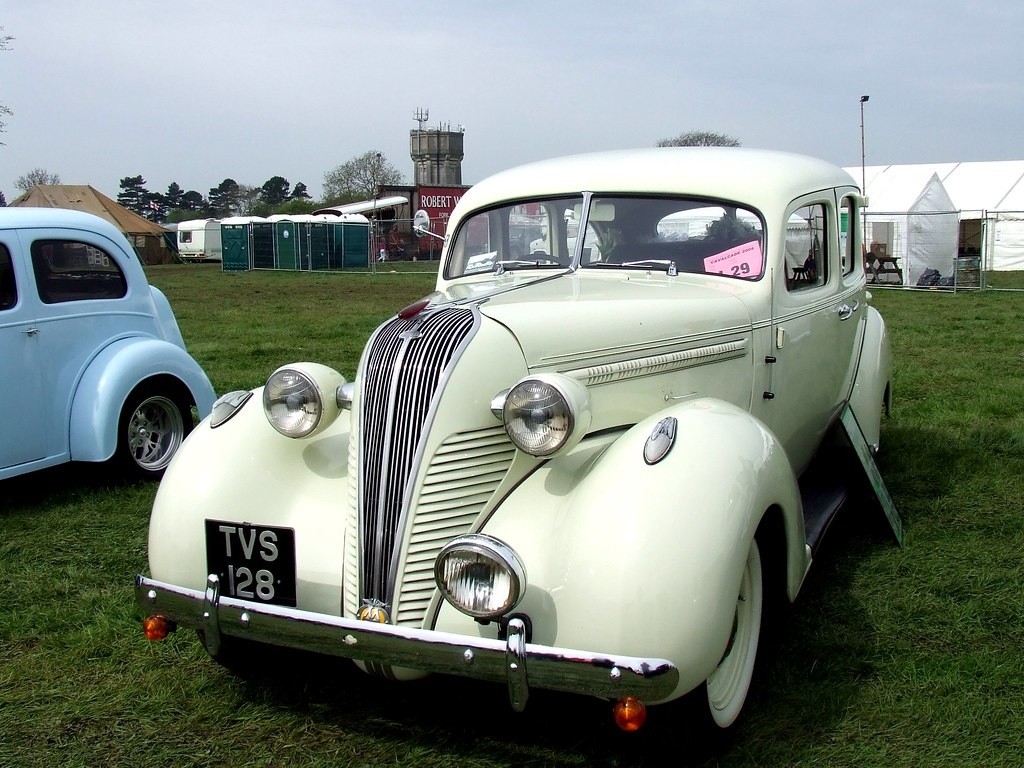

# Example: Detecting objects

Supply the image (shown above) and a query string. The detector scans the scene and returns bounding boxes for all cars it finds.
[0,206,218,492]
[134,146,897,760]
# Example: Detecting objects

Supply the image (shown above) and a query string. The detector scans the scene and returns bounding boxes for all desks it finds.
[842,256,903,284]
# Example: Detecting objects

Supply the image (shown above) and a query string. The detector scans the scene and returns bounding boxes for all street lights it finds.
[860,93,870,277]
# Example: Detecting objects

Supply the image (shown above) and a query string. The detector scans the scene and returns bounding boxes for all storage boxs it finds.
[872,241,886,257]
